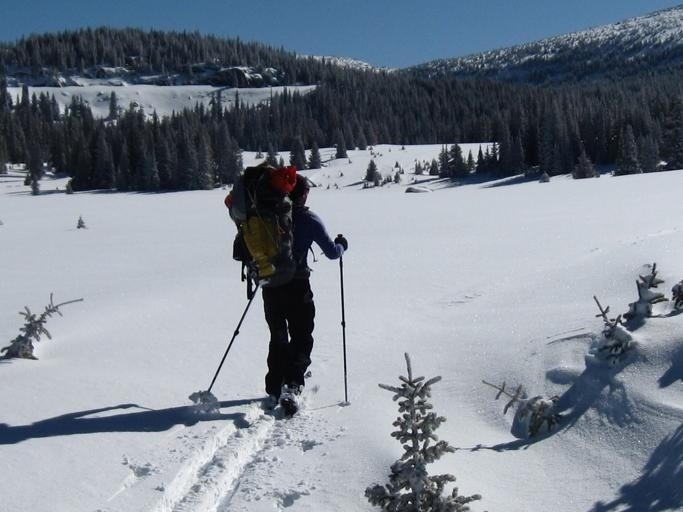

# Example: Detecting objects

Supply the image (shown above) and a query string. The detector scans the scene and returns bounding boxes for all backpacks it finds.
[223,164,296,287]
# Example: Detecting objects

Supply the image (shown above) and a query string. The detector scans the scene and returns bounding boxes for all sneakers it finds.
[280,384,299,414]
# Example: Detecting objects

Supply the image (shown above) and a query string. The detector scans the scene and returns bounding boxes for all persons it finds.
[225,167,348,413]
